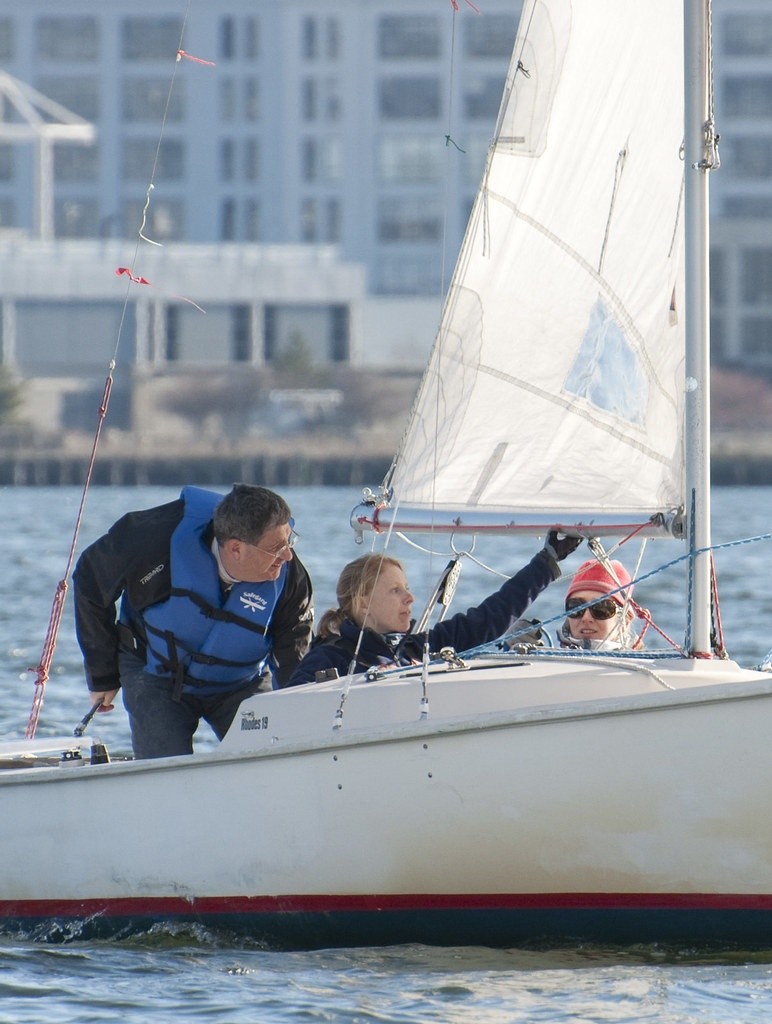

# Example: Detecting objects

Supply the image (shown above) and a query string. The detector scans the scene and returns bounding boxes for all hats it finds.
[566,558,634,614]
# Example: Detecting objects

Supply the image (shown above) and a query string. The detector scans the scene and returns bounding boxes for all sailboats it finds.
[3,2,772,966]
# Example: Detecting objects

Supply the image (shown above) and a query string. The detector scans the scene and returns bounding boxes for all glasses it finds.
[231,528,301,558]
[566,598,615,620]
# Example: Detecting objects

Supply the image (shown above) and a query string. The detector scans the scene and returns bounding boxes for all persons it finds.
[72,483,315,759]
[502,557,647,653]
[288,526,584,687]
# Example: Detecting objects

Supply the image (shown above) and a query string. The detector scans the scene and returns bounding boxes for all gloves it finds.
[544,520,595,562]
